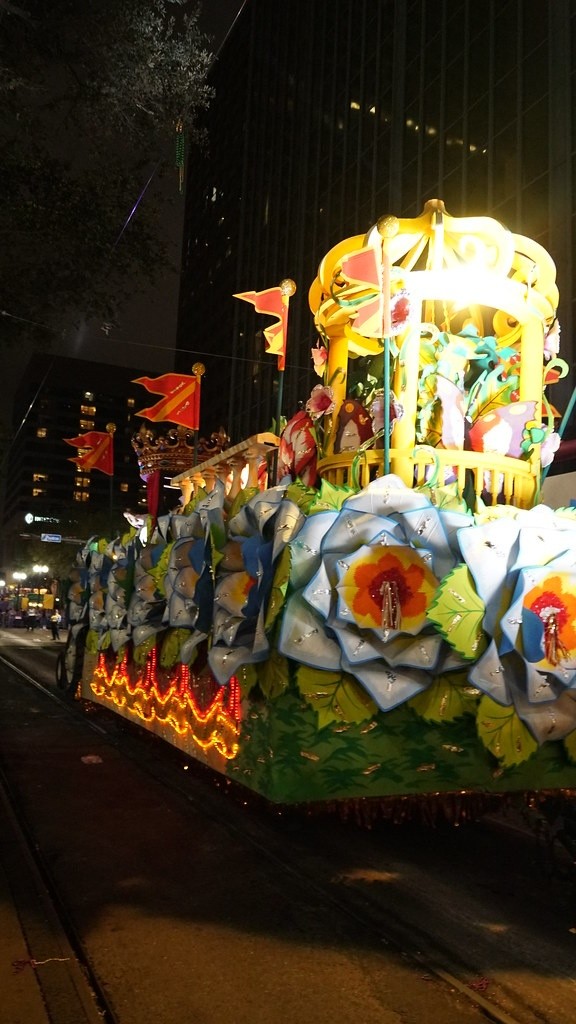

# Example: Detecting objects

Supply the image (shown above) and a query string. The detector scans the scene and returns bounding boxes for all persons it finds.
[50,609,61,640]
[1,607,37,631]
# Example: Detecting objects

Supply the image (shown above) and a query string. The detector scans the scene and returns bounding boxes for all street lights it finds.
[33,565,48,610]
[14,573,26,601]
